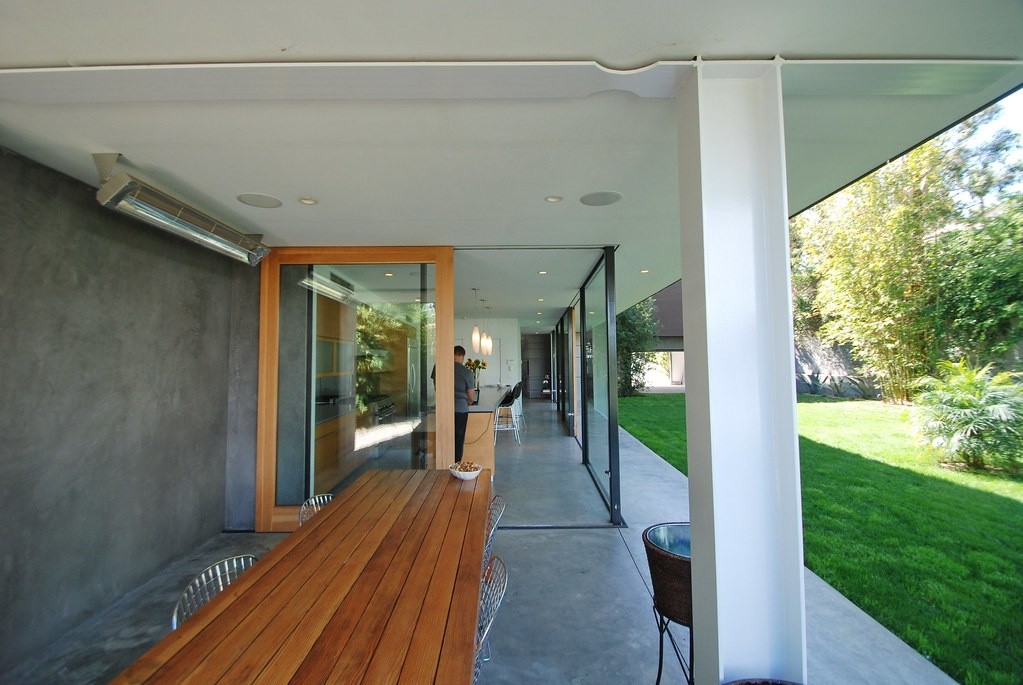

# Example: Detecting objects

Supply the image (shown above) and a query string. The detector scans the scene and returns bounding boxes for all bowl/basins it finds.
[448,464,482,481]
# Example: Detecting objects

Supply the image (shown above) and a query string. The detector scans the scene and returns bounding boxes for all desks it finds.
[107,464,493,685]
[426,386,509,476]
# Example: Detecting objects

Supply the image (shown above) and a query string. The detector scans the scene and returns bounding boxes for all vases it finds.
[469,368,481,390]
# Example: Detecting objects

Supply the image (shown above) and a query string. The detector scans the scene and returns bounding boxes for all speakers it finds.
[95,171,138,207]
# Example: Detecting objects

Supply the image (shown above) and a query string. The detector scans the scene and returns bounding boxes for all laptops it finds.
[471,389,480,405]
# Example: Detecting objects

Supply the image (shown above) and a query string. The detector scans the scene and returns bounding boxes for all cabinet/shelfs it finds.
[314,294,357,481]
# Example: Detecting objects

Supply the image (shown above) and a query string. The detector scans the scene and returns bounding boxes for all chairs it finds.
[299,494,334,529]
[483,493,506,571]
[171,554,258,633]
[473,555,509,684]
[493,381,527,447]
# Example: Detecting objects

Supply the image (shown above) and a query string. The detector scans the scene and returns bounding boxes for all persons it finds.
[431,345,474,464]
[542,374,550,399]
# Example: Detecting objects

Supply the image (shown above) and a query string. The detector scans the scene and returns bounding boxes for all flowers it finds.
[465,358,488,388]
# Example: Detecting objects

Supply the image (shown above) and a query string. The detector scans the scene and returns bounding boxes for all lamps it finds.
[92,153,274,268]
[470,287,494,356]
[294,272,356,306]
